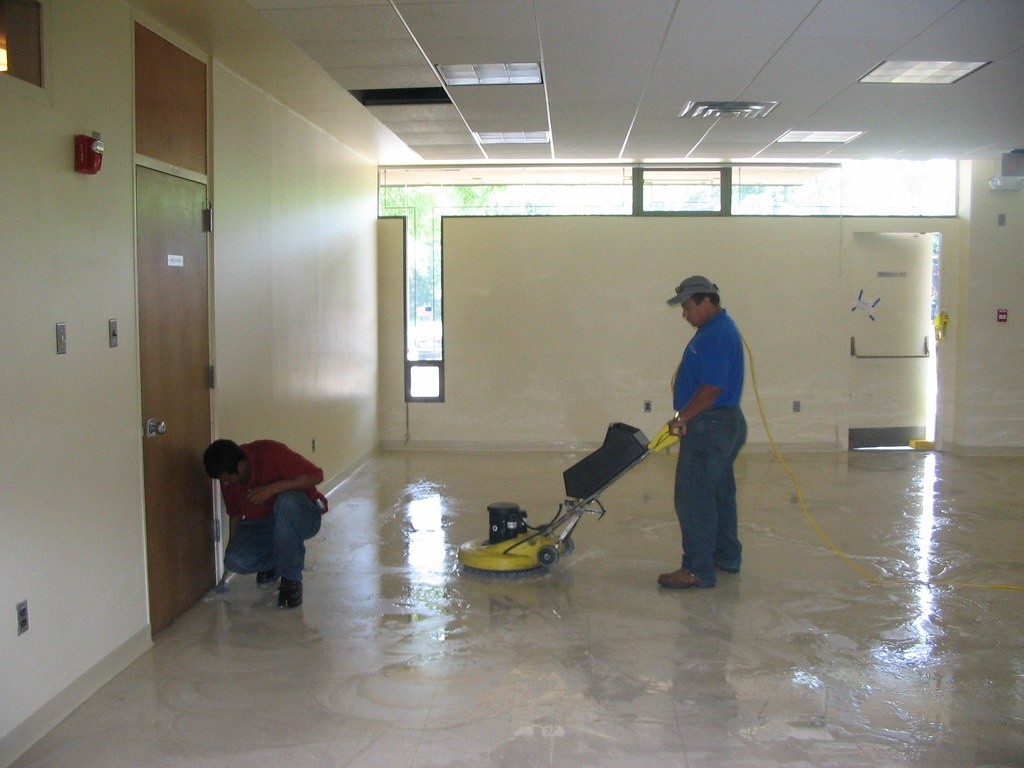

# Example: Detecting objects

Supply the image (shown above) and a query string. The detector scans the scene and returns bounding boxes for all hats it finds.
[666,275,716,305]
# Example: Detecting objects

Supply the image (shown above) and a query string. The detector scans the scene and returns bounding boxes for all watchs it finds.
[675,411,680,422]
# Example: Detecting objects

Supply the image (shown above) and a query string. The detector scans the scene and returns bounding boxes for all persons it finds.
[655,276,748,591]
[203,439,329,607]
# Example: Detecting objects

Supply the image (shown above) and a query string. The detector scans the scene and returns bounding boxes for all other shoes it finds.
[256,570,278,583]
[277,576,303,607]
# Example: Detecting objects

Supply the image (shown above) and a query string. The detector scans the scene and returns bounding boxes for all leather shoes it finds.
[657,569,715,589]
